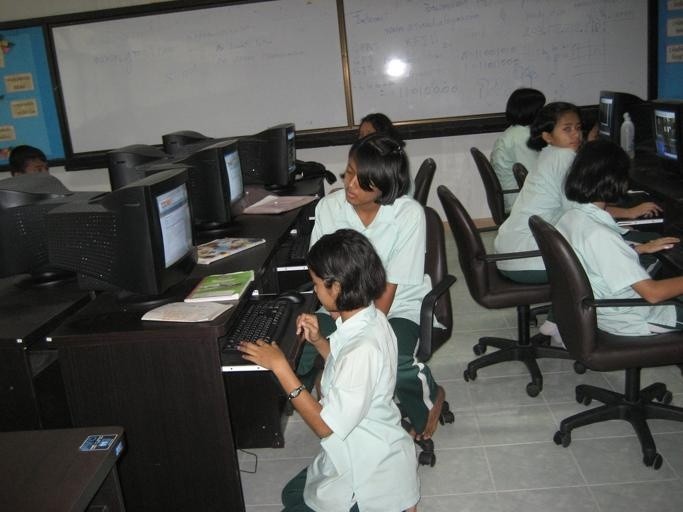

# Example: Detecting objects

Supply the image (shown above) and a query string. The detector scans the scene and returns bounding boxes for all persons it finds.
[286,131,445,441]
[494,101,664,350]
[329,113,416,200]
[554,140,683,337]
[9,145,49,178]
[235,228,421,511]
[490,88,546,214]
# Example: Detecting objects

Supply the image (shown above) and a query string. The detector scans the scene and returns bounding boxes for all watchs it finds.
[286,384,307,404]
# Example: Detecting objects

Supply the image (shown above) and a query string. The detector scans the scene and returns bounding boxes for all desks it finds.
[0,425,127,512]
[0,276,96,439]
[44,176,325,512]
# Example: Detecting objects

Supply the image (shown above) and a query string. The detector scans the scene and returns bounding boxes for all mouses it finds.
[639,209,664,220]
[276,289,304,304]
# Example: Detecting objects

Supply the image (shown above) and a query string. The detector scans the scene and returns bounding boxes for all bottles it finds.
[620,112,635,159]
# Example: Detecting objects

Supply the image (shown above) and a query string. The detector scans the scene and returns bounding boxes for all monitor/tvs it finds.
[162,130,214,155]
[0,172,110,289]
[651,99,683,175]
[599,90,652,146]
[145,138,245,234]
[47,168,198,308]
[107,144,174,192]
[241,123,296,195]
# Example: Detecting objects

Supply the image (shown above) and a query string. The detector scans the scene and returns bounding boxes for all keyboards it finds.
[220,300,293,355]
[656,243,683,274]
[288,232,311,260]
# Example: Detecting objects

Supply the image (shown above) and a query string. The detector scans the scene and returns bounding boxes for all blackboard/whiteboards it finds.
[42,0,658,171]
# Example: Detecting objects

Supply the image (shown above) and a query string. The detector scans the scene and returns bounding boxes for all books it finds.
[243,194,320,214]
[193,237,266,264]
[184,269,255,303]
[616,218,664,227]
